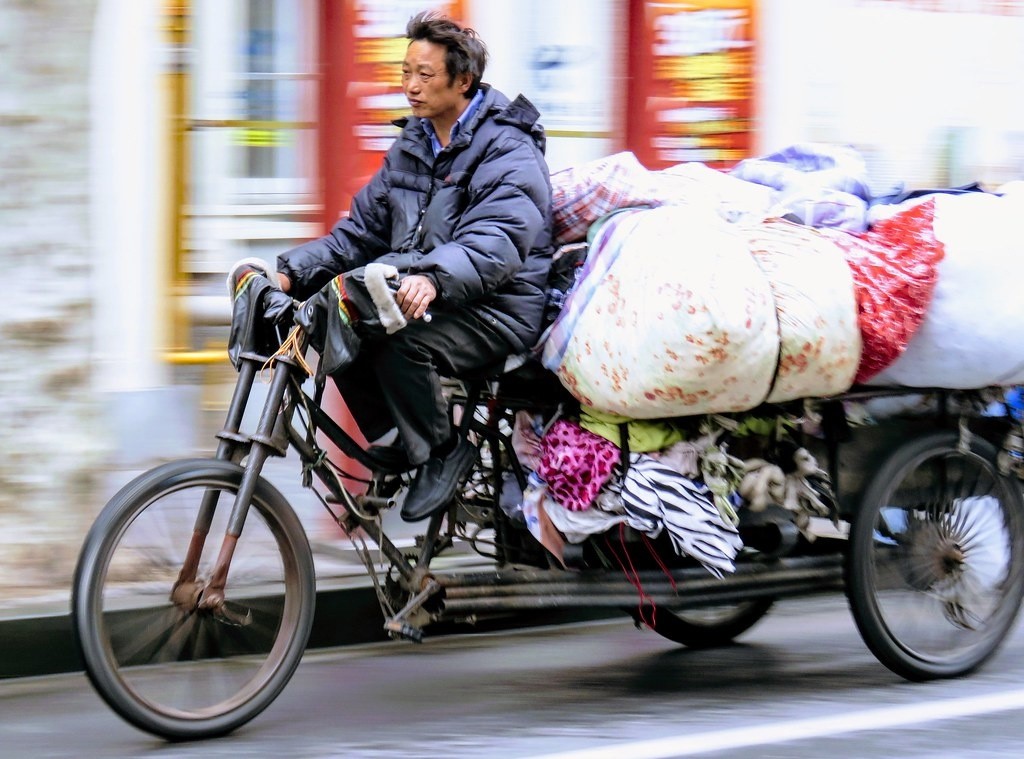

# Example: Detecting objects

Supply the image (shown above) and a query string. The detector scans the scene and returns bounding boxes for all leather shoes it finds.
[401,431,478,522]
[366,423,430,469]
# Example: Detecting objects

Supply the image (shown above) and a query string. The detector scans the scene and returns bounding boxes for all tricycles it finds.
[72,261,1024,746]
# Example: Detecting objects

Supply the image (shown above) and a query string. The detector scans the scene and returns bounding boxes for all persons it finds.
[273,9,553,530]
